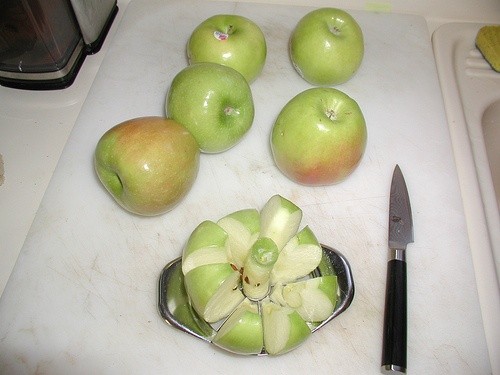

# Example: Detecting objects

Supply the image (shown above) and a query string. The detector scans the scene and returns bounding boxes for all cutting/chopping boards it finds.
[0,0,494,375]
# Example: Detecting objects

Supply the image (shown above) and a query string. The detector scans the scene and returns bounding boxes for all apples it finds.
[183,262,245,324]
[214,209,258,265]
[263,301,311,355]
[210,303,262,355]
[91,115,203,220]
[270,85,369,187]
[181,220,234,275]
[287,6,363,85]
[184,14,266,83]
[163,63,255,152]
[268,225,323,284]
[241,238,278,299]
[275,276,339,324]
[259,194,302,251]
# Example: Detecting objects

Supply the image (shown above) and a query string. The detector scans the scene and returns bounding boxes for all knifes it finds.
[379,164,415,375]
